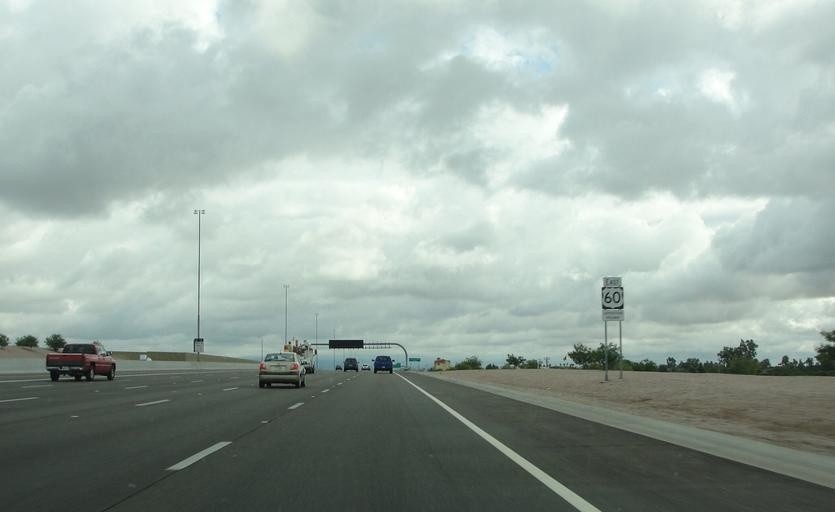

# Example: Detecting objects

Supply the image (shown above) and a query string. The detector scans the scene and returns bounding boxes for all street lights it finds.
[314,312,319,349]
[191,207,206,360]
[282,284,290,345]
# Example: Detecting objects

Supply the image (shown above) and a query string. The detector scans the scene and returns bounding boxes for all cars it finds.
[361,364,370,370]
[256,351,306,389]
[335,364,343,371]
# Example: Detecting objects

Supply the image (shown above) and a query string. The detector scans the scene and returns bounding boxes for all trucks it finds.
[283,337,318,374]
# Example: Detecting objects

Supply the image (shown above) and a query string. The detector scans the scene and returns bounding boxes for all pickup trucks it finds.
[45,339,117,382]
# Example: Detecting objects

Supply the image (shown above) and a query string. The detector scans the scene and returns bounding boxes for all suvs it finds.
[343,358,360,372]
[371,356,395,373]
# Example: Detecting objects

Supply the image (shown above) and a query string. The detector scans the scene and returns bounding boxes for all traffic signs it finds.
[602,288,623,311]
[409,358,420,361]
